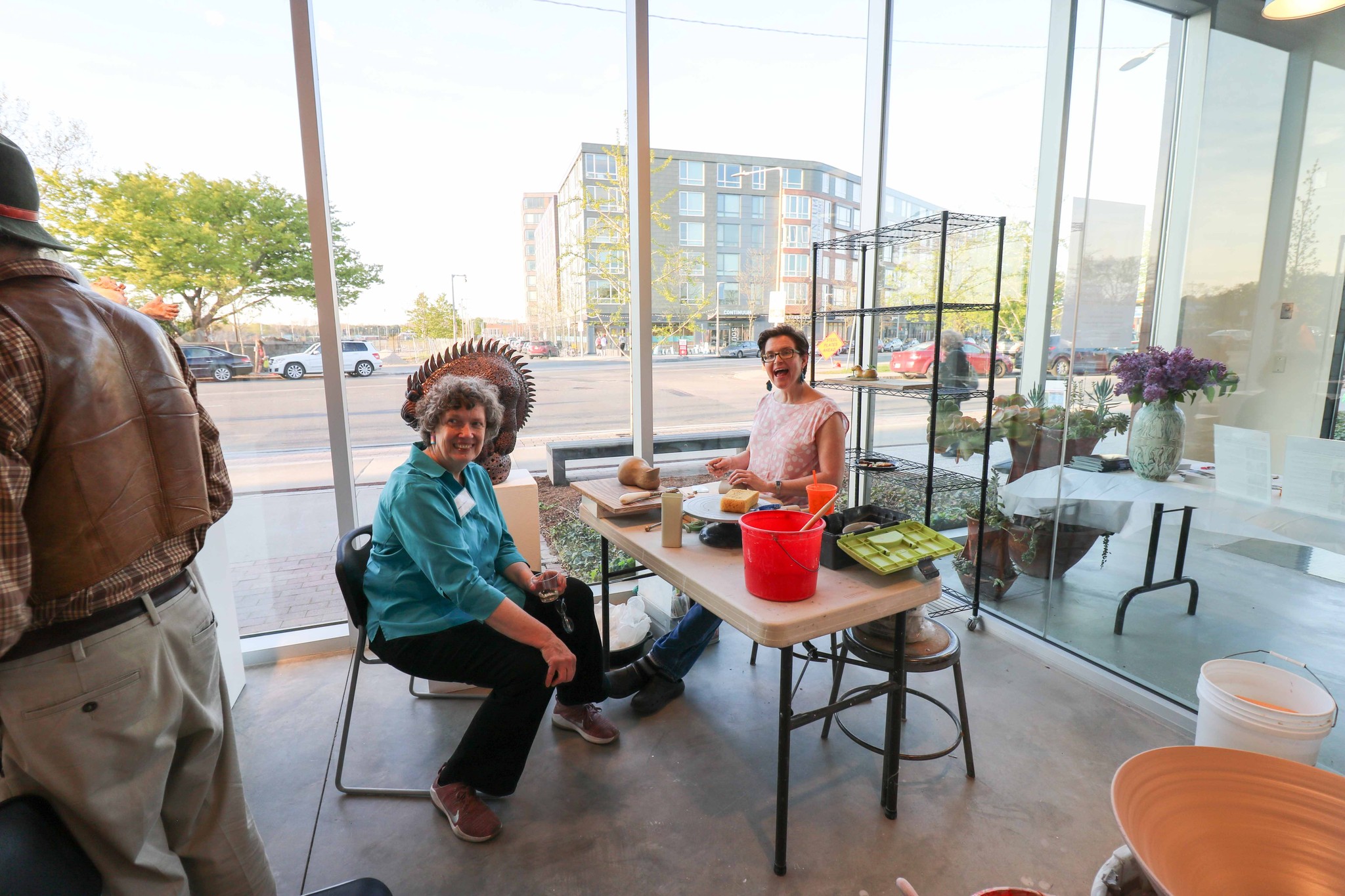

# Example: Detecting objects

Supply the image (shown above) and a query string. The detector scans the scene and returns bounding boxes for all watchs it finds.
[775,480,781,495]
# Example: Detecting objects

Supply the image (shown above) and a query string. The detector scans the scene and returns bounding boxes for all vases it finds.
[1129,400,1187,482]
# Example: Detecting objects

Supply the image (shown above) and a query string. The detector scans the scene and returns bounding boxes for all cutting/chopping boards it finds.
[569,477,690,514]
[824,377,942,391]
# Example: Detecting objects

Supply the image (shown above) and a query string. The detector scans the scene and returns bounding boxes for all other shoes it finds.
[604,663,648,699]
[630,675,685,712]
[256,372,260,374]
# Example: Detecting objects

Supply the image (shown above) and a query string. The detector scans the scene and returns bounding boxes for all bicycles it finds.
[555,346,577,357]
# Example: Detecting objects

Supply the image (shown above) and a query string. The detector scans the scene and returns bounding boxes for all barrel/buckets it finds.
[1194,649,1339,767]
[738,510,826,603]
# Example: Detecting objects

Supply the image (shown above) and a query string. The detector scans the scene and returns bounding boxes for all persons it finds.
[0,136,279,896]
[361,374,620,841]
[595,334,626,357]
[604,326,850,713]
[89,276,179,320]
[939,330,971,457]
[256,340,267,374]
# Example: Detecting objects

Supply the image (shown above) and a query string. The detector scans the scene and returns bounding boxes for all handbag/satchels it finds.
[263,358,269,368]
[596,344,602,349]
[965,362,979,391]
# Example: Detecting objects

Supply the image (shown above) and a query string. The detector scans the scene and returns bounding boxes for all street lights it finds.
[716,282,728,357]
[823,293,833,340]
[451,274,468,345]
[731,166,784,328]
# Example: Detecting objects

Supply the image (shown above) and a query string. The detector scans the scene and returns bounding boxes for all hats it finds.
[0,132,76,252]
[603,334,606,337]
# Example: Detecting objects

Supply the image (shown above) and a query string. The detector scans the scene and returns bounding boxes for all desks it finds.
[992,456,1345,636]
[423,468,542,696]
[576,490,942,877]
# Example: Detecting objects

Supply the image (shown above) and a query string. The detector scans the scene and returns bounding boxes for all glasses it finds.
[760,349,801,362]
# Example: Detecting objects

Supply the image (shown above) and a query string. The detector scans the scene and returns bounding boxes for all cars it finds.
[177,345,254,381]
[505,337,532,354]
[963,334,1140,377]
[877,338,920,353]
[808,340,856,356]
[890,340,1014,380]
[719,341,762,359]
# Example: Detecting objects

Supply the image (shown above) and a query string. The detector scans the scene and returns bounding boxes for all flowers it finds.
[1113,347,1238,405]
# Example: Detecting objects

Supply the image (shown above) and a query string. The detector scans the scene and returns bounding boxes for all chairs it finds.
[333,523,515,803]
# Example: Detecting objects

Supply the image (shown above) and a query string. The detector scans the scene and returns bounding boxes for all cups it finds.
[806,483,837,516]
[660,493,684,548]
[530,570,559,603]
[972,886,1052,896]
[672,589,689,617]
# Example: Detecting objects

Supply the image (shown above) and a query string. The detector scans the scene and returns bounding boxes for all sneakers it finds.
[551,694,620,744]
[431,768,504,842]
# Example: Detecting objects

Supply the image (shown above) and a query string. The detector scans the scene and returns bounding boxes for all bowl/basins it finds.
[609,629,654,670]
[1110,745,1345,896]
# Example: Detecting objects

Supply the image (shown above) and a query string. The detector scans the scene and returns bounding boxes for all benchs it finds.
[544,426,752,487]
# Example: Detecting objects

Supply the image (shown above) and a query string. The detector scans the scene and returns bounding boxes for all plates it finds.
[851,459,904,471]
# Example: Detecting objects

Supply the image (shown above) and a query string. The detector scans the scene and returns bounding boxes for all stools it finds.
[821,612,976,804]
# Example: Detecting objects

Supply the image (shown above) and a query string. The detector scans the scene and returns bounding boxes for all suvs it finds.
[529,340,559,359]
[269,340,384,380]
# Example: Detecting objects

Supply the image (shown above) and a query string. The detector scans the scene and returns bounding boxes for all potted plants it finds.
[930,378,1129,608]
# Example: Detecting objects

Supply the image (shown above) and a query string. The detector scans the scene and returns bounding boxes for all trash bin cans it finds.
[556,341,562,349]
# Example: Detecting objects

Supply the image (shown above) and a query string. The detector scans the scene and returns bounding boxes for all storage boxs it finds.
[818,504,965,577]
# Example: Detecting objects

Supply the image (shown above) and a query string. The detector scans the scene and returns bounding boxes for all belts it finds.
[0,568,194,663]
